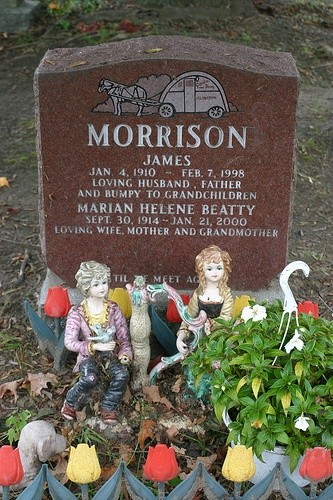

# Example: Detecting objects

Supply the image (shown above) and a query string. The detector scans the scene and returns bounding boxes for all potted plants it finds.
[182,260,333,487]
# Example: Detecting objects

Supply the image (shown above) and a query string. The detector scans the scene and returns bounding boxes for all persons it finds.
[175,245,234,409]
[61,260,133,425]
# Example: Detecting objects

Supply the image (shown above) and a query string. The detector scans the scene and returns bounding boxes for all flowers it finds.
[146,296,189,354]
[124,443,202,500]
[201,445,278,500]
[0,445,46,500]
[278,445,333,500]
[46,444,124,500]
[23,287,82,368]
